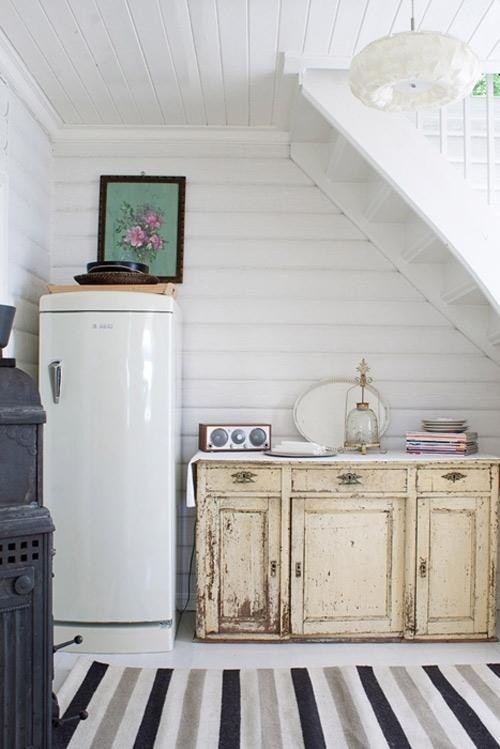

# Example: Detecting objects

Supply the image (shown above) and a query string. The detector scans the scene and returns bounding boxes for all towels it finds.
[272,441,326,455]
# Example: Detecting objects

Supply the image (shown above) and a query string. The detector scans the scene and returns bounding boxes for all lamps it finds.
[347,0,481,111]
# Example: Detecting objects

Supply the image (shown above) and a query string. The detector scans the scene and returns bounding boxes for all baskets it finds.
[74,272,160,284]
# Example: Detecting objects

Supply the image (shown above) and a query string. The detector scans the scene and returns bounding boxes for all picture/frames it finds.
[96,174,187,285]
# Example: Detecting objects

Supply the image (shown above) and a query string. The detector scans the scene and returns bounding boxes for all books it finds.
[404,428,481,458]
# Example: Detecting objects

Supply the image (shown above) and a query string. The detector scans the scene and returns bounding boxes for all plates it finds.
[72,272,160,286]
[265,446,335,458]
[421,417,468,434]
[291,376,391,452]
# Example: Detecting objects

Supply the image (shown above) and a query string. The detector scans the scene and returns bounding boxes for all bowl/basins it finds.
[85,260,150,276]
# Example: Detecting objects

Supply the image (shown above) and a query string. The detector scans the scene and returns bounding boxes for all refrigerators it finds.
[37,294,178,658]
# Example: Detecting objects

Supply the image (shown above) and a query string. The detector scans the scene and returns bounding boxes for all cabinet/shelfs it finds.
[293,469,406,635]
[207,469,280,634]
[415,469,491,635]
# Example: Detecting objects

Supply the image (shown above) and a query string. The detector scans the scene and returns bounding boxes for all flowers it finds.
[113,203,167,264]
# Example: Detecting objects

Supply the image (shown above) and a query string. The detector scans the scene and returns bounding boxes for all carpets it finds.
[56,661,500,749]
